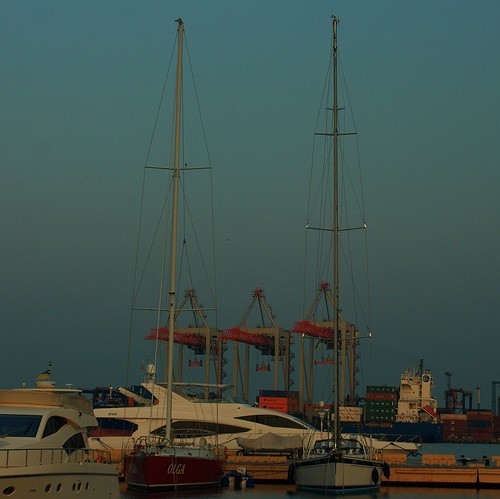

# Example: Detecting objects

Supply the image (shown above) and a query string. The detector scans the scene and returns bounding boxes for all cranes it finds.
[147,283,359,413]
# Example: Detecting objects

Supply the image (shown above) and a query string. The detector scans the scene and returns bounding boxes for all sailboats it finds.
[287,15,389,492]
[125,16,229,490]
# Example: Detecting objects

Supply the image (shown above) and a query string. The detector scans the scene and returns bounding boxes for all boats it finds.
[0,368,121,499]
[90,384,420,455]
[257,357,443,443]
